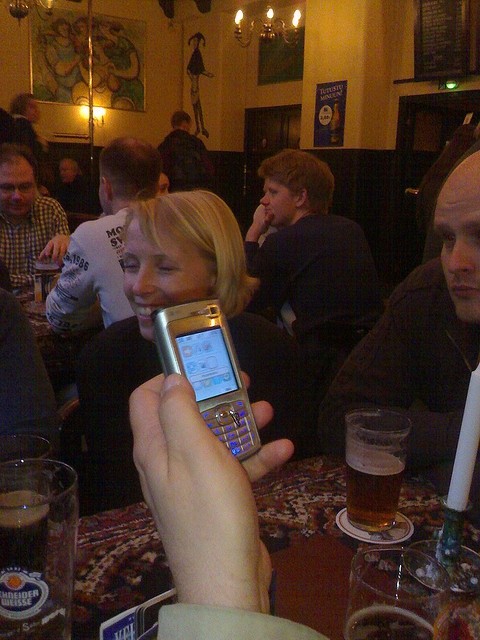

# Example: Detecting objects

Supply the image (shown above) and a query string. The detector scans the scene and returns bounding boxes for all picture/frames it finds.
[27,4,147,113]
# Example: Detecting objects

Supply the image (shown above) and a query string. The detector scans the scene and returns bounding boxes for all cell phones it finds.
[150,297,263,464]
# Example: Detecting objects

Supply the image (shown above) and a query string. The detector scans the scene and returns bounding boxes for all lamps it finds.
[233,0,302,47]
[7,0,54,26]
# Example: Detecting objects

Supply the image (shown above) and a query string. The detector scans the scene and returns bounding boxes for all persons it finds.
[155,172,172,195]
[49,136,159,331]
[2,257,63,489]
[57,157,96,210]
[317,149,480,471]
[157,111,216,189]
[123,364,295,640]
[34,18,144,110]
[5,92,41,146]
[245,148,386,368]
[69,190,300,500]
[4,144,71,295]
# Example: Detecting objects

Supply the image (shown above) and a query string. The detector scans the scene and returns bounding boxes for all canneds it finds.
[32,261,63,306]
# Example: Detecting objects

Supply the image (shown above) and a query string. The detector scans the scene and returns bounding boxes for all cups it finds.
[344,407,413,534]
[0,456,80,639]
[0,434,53,582]
[392,536,480,640]
[342,544,452,640]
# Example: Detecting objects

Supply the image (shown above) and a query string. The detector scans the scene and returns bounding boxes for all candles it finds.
[403,362,479,594]
